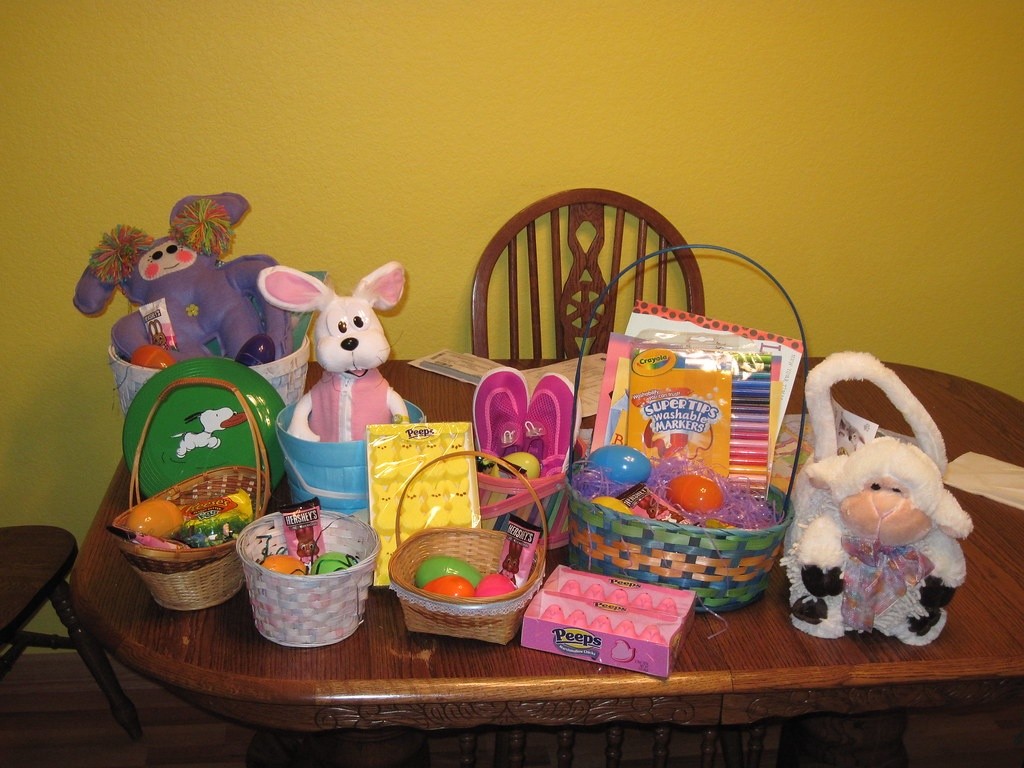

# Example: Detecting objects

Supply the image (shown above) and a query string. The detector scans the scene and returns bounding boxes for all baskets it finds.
[565,244,809,614]
[388,451,547,645]
[235,510,381,647]
[107,377,271,610]
[476,436,588,549]
[107,336,310,415]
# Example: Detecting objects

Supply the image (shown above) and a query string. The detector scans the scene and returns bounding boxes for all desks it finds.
[68,357,1024,766]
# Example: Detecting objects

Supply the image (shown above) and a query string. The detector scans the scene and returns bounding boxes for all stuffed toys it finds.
[73,193,294,370]
[780,350,974,646]
[257,261,410,443]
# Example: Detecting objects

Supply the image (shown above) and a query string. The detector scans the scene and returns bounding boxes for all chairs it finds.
[0,522,149,742]
[470,186,704,356]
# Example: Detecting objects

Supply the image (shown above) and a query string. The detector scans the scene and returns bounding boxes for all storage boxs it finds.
[518,562,697,677]
